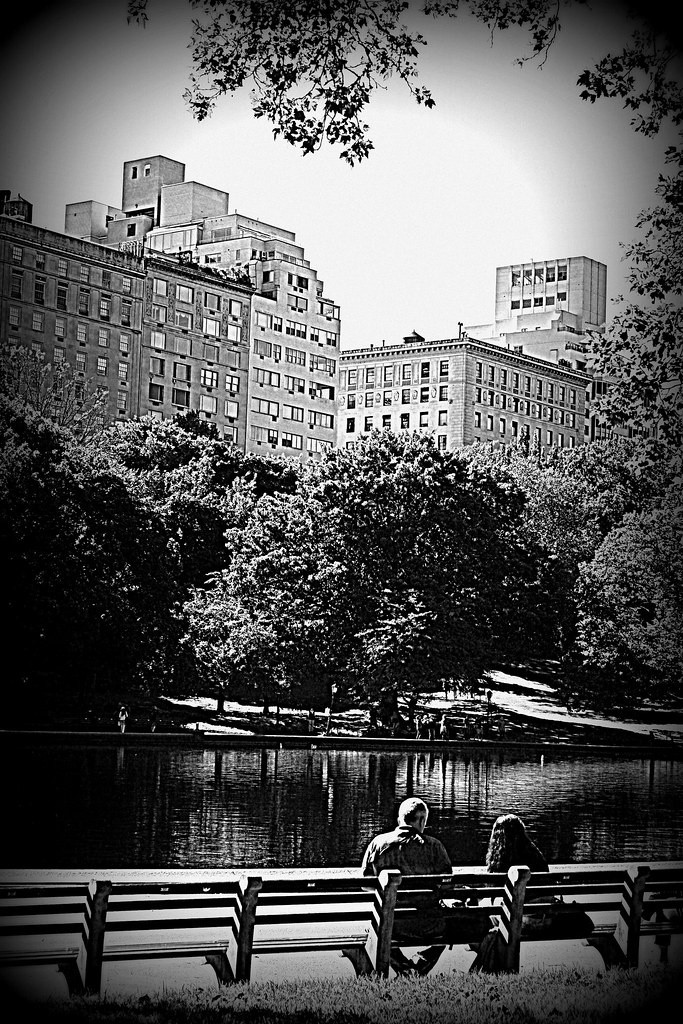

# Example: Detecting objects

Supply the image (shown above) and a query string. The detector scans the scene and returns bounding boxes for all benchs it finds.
[0,867,683,1001]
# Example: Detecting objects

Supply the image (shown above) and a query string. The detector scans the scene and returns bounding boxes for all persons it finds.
[468,815,549,975]
[147,706,159,733]
[308,708,314,733]
[118,706,128,733]
[414,713,506,740]
[359,798,455,976]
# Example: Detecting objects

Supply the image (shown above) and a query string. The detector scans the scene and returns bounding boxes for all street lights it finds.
[325,684,339,736]
[487,690,493,716]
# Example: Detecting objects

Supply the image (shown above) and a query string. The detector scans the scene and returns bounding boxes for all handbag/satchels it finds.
[542,894,595,935]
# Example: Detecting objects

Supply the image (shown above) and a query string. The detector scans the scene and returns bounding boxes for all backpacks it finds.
[468,929,508,977]
